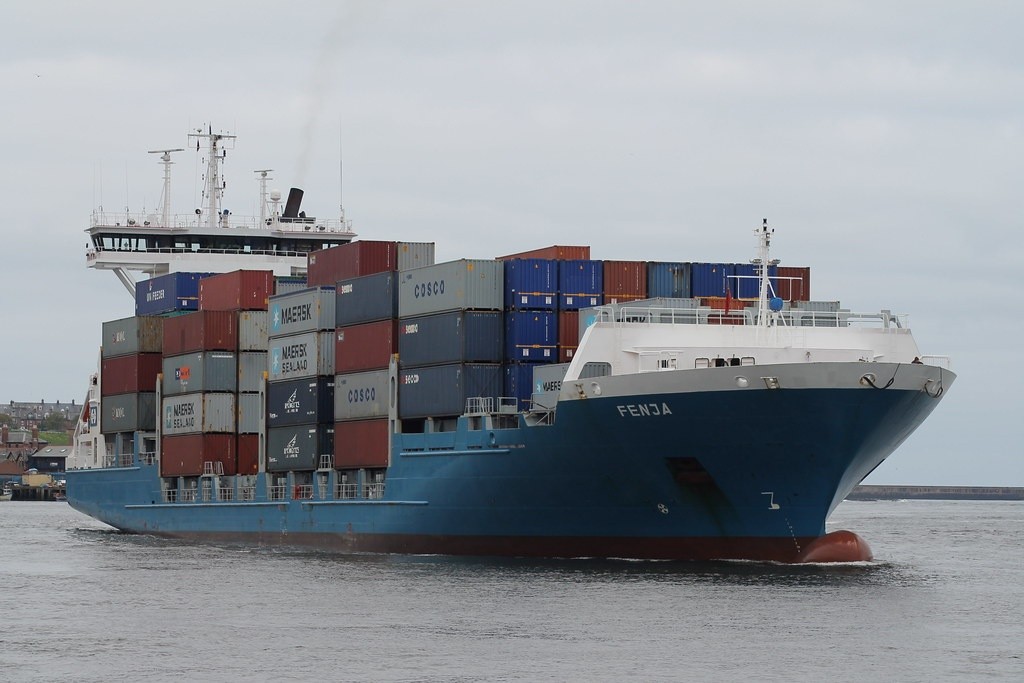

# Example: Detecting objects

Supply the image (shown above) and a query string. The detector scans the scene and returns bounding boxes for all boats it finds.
[64,121,957,565]
